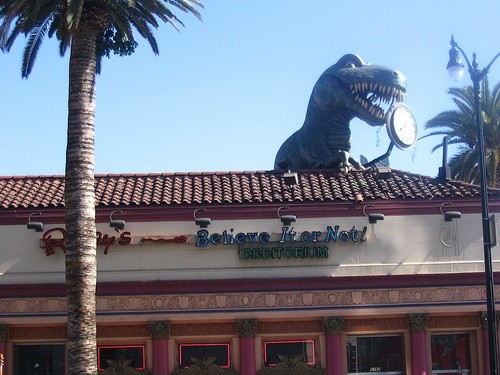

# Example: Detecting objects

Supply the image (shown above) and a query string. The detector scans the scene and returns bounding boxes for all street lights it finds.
[445,36,499,374]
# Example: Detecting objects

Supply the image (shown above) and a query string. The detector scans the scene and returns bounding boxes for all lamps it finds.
[362,203,384,224]
[108,209,126,230]
[276,205,298,227]
[193,207,212,229]
[26,211,45,232]
[439,198,463,223]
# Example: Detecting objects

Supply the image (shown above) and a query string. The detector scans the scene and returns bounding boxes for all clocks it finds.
[391,104,419,147]
